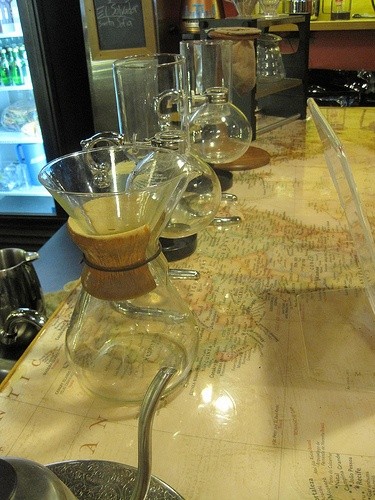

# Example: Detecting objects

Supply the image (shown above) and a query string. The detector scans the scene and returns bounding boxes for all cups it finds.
[113,52,190,141]
[178,39,235,99]
[15,163,29,191]
[232,0,352,20]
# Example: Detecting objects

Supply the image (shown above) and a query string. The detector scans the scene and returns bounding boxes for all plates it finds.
[44,461,186,500]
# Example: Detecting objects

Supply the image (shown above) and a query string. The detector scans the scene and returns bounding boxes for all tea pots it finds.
[1,366,179,499]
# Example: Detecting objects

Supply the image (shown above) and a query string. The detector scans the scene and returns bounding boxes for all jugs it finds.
[16,144,47,186]
[0,248,48,344]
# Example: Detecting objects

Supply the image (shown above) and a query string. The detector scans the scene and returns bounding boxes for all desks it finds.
[0,104,375,500]
[182,18,375,33]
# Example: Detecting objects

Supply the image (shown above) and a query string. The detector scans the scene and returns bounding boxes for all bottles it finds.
[0,39,31,86]
[39,148,211,401]
[255,32,286,83]
[80,131,222,239]
[153,86,253,165]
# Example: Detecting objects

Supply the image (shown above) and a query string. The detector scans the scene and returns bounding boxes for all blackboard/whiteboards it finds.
[83,0,158,62]
